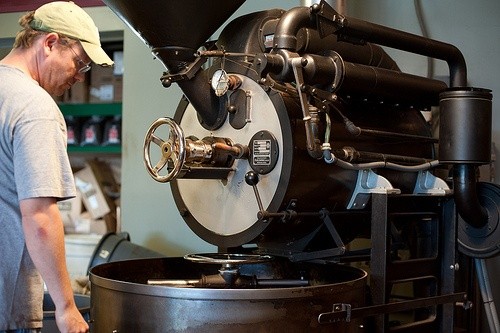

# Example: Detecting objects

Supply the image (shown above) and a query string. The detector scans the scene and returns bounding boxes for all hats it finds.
[30,1,117,69]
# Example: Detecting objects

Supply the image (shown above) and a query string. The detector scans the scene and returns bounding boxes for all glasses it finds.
[59,34,92,73]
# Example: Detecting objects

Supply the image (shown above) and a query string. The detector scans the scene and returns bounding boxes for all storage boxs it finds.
[58,157,116,234]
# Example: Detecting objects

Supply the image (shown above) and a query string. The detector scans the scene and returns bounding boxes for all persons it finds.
[0,0,115,333]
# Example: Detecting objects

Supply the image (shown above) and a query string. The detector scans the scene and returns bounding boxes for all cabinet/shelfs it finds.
[0,29,123,243]
